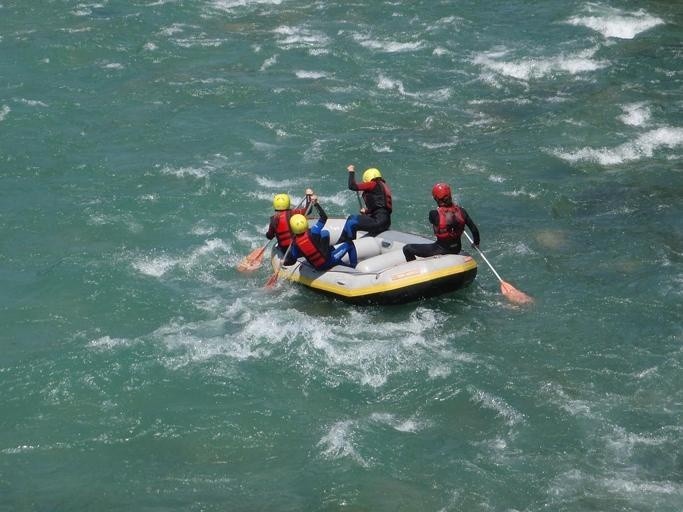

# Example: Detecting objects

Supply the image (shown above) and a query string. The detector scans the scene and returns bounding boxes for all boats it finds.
[270,215,479,306]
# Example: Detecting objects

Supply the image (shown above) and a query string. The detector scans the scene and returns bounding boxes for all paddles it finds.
[263,197,315,290]
[464,230,531,304]
[237,192,307,274]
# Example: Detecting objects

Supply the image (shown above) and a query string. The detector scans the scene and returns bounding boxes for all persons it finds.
[402,182,480,264]
[344,164,393,240]
[266,187,314,258]
[279,195,358,271]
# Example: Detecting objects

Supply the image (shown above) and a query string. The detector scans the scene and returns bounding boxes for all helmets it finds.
[289,214,308,234]
[273,193,290,210]
[362,168,382,182]
[432,183,451,200]
